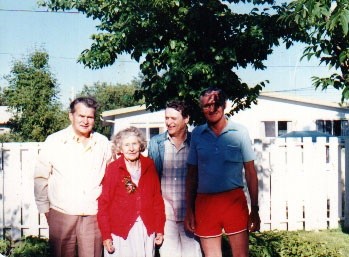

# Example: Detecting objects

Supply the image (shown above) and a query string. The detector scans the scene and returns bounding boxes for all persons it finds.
[34,97,120,257]
[148,101,205,257]
[184,86,261,257]
[97,126,166,257]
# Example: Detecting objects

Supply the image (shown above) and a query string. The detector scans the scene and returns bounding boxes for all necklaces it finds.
[125,160,139,175]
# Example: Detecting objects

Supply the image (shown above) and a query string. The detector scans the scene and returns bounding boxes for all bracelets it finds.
[251,205,259,211]
